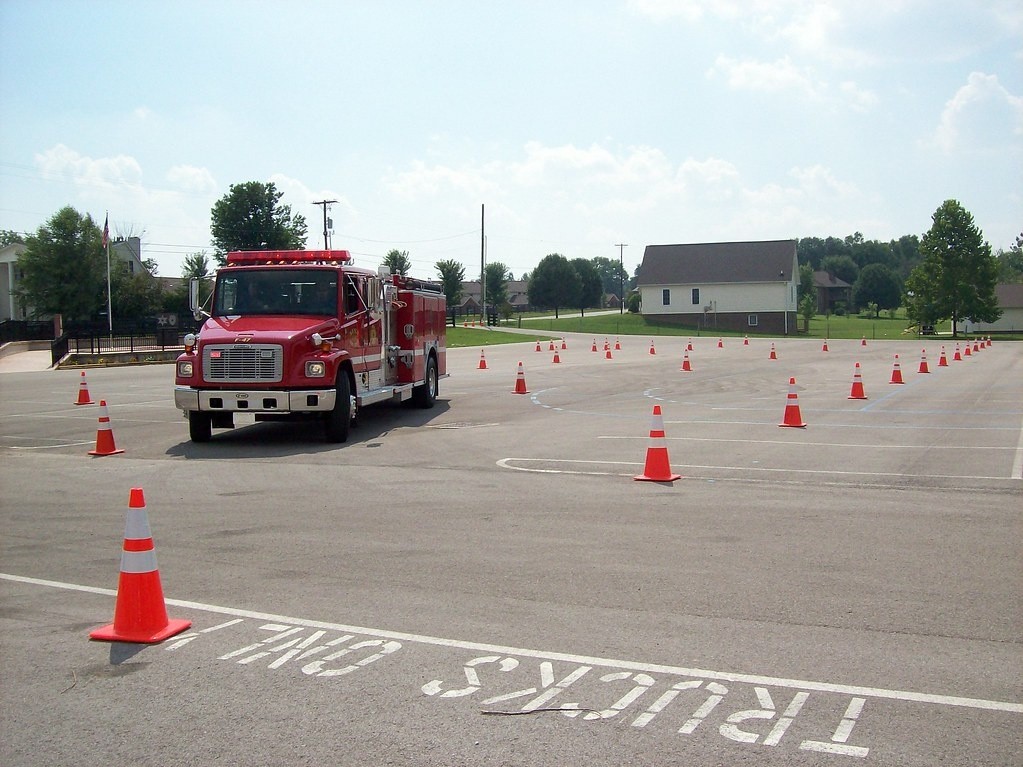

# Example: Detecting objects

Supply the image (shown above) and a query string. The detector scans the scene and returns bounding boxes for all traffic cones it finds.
[889,354,905,384]
[847,362,868,400]
[778,377,807,428]
[89,489,192,644]
[685,337,694,351]
[632,406,681,482]
[680,348,694,372]
[591,336,621,359]
[717,337,724,348]
[744,334,749,345]
[952,336,992,360]
[821,337,829,352]
[463,318,484,327]
[74,371,95,405]
[476,348,489,369]
[648,340,656,354]
[917,348,931,373]
[938,345,949,366]
[511,360,530,394]
[861,335,866,345]
[87,399,125,456]
[535,336,568,363]
[768,342,778,359]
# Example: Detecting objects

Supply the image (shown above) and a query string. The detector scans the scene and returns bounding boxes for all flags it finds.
[102,215,108,248]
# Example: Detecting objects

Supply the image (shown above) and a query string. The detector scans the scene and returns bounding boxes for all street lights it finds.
[614,243,628,314]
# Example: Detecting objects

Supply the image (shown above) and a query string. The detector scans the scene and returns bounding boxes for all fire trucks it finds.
[173,251,452,442]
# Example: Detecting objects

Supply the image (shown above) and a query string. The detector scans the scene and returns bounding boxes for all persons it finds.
[235,276,279,308]
[299,279,337,308]
